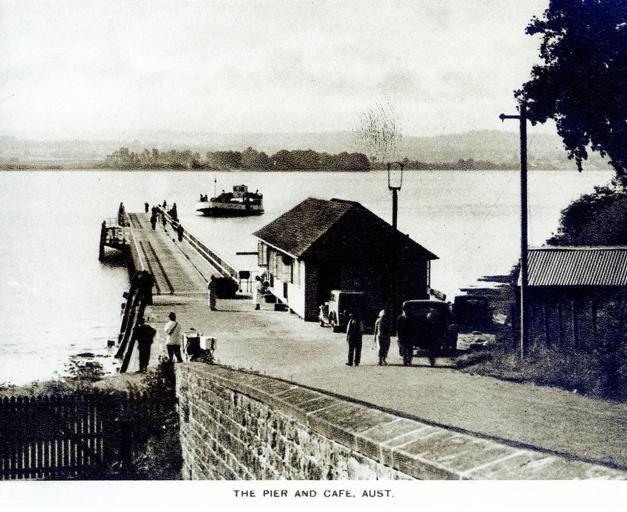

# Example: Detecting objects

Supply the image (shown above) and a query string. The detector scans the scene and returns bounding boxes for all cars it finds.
[319,291,372,332]
[398,300,458,356]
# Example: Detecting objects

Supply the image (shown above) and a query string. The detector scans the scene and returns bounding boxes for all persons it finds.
[424,309,442,367]
[346,312,365,366]
[254,275,264,310]
[207,276,217,311]
[164,312,183,363]
[374,309,392,365]
[135,318,156,373]
[145,200,184,242]
[396,304,417,367]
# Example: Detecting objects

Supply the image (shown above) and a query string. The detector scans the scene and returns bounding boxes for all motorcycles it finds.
[186,328,213,363]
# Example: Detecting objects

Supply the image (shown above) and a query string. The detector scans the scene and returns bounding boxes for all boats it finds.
[196,184,265,218]
